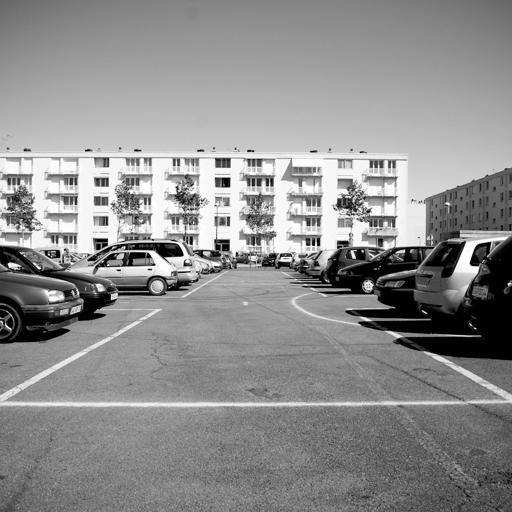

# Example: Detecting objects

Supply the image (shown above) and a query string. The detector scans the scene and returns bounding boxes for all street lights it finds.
[443,201,452,240]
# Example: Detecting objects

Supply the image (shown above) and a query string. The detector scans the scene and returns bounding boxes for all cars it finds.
[290,246,338,285]
[326,245,405,286]
[335,245,435,295]
[275,253,294,268]
[0,264,85,343]
[462,234,511,350]
[0,245,119,313]
[415,237,510,317]
[262,252,278,268]
[66,238,233,295]
[374,269,417,306]
